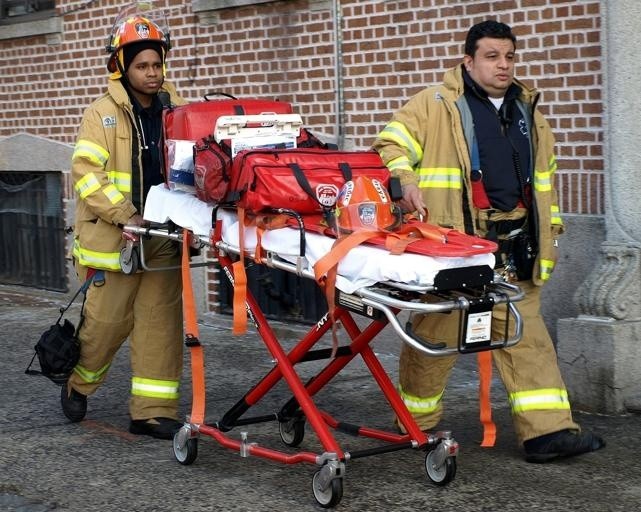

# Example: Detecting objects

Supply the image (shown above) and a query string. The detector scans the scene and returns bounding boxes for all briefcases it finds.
[213,112,305,150]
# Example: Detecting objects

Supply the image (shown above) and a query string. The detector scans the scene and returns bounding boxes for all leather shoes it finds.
[60,385,86,423]
[128,415,189,439]
[522,430,608,464]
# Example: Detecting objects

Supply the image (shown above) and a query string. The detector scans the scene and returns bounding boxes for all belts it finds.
[477,218,526,236]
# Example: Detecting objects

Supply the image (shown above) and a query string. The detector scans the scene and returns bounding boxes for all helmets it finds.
[336,177,396,232]
[106,2,173,64]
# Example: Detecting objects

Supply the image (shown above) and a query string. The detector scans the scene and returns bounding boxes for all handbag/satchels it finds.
[26,314,83,386]
[166,135,196,184]
[232,150,391,216]
[160,99,294,186]
[194,129,340,202]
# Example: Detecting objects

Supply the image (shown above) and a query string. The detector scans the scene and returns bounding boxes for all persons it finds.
[369,19,609,464]
[60,15,188,441]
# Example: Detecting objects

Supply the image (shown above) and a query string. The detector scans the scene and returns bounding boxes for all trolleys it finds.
[123,218,532,504]
